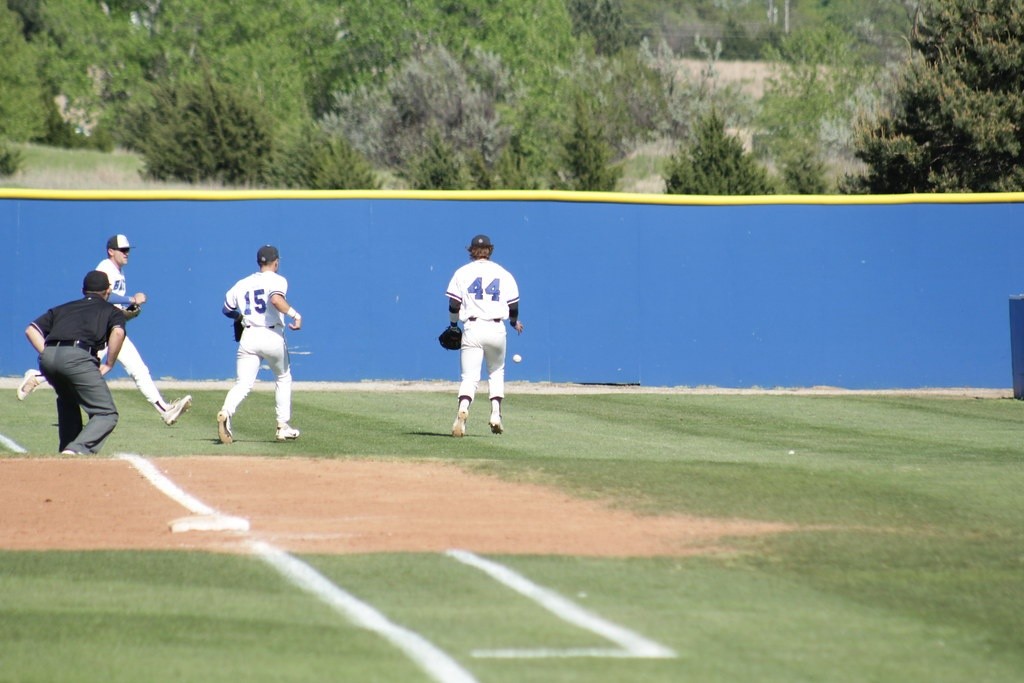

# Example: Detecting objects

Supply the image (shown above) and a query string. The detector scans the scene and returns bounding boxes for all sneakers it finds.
[489,415,505,434]
[160,394,193,425]
[275,425,300,442]
[17,369,39,401]
[217,412,233,444]
[452,408,469,437]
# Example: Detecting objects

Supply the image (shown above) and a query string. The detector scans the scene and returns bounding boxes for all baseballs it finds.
[513,354,522,363]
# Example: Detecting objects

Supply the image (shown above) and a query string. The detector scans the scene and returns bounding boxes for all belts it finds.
[470,317,501,322]
[47,339,96,355]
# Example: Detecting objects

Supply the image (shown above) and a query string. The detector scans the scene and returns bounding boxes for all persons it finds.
[217,244,303,443]
[24,270,125,456]
[16,235,193,426]
[448,235,523,439]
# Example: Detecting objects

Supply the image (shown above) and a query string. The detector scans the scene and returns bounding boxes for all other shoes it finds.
[63,449,82,456]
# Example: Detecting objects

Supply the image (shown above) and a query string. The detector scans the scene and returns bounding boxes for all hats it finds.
[472,234,491,246]
[83,270,112,292]
[256,245,282,263]
[107,235,137,249]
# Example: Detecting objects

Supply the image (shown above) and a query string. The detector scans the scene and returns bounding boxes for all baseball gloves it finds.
[234,320,244,342]
[121,304,141,321]
[438,326,462,350]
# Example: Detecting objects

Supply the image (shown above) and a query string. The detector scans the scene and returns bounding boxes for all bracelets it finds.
[450,321,458,327]
[133,296,136,304]
[286,306,297,317]
[510,321,516,326]
